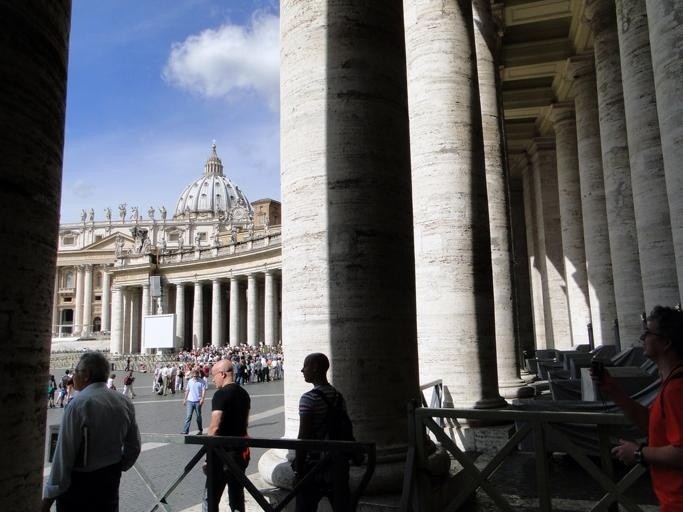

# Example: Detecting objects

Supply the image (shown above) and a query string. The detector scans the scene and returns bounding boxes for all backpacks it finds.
[311,388,356,463]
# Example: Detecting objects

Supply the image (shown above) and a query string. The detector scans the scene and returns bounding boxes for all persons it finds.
[201,359,250,512]
[77,202,269,257]
[178,369,205,435]
[41,351,140,512]
[47,342,283,409]
[287,352,356,511]
[585,303,682,510]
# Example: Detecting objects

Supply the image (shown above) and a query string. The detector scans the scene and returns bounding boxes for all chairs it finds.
[524,344,659,400]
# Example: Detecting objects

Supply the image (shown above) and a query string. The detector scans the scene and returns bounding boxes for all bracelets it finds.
[634,444,643,461]
